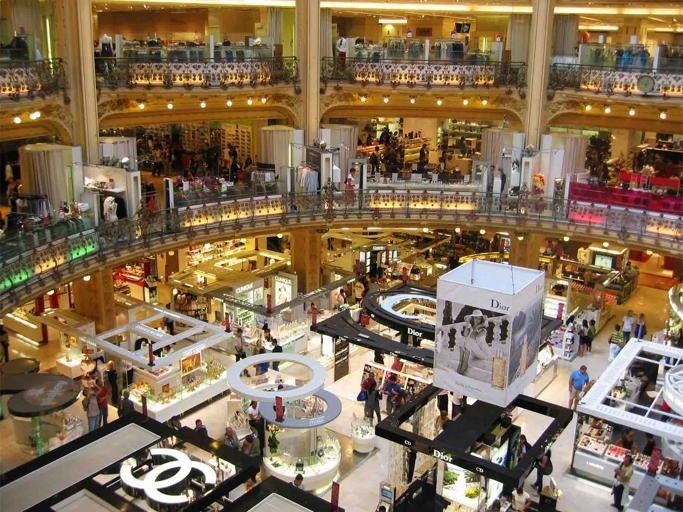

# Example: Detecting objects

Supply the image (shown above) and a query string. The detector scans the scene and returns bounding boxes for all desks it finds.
[351,427,376,454]
[56,355,82,381]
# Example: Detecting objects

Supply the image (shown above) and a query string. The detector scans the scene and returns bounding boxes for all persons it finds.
[0,30,683,512]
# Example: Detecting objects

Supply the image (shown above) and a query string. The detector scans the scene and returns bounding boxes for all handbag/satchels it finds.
[542,461,552,475]
[82,399,89,411]
[578,327,584,337]
[118,408,128,417]
[391,394,409,406]
[357,391,368,401]
[369,156,378,164]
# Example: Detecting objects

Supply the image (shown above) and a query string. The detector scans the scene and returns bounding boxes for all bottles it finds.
[520,334,528,375]
[490,348,507,389]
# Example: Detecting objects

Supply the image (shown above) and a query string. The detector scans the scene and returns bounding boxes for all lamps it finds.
[585,103,668,120]
[14,113,22,124]
[138,96,267,109]
[359,94,489,106]
[29,110,41,121]
[514,232,529,241]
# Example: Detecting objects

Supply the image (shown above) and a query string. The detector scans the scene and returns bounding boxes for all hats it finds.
[464,310,488,327]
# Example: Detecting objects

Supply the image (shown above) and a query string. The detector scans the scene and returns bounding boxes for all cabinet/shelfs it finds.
[242,320,308,371]
[357,137,431,172]
[219,278,264,327]
[143,121,253,164]
[448,121,489,143]
[552,324,580,361]
